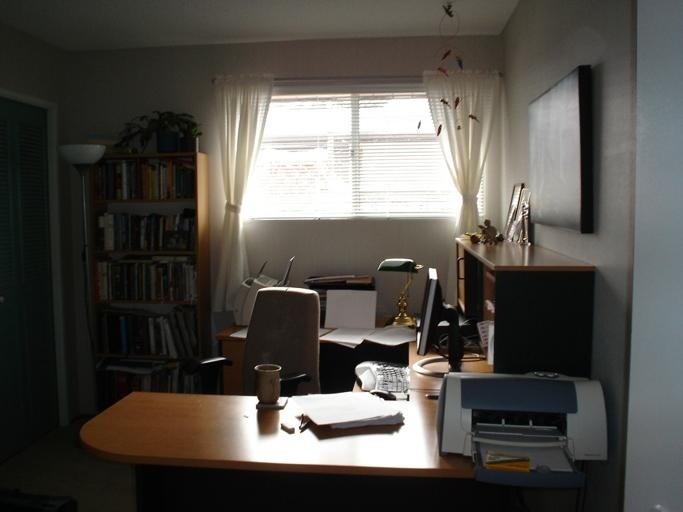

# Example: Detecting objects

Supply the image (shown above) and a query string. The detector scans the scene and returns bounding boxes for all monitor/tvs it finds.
[412,268,464,377]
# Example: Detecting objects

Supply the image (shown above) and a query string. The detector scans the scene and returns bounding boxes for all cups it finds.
[253,363,282,403]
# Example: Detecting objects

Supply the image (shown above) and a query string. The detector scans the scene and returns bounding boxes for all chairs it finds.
[240,287,321,396]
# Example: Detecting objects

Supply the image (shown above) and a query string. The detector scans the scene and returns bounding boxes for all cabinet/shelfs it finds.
[89,152,211,412]
[454,235,596,383]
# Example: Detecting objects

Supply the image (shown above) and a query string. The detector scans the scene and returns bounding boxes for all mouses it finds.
[370,390,396,400]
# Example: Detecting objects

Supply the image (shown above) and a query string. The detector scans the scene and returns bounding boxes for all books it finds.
[291,391,405,429]
[90,158,199,411]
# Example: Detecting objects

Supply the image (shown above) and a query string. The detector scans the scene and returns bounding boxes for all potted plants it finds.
[120,109,203,153]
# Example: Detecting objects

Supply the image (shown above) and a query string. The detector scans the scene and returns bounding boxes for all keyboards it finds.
[355,360,410,393]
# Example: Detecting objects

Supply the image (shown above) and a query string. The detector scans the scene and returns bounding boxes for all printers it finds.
[435,372,613,490]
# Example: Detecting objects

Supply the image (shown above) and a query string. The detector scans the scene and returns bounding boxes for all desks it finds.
[79,321,516,512]
